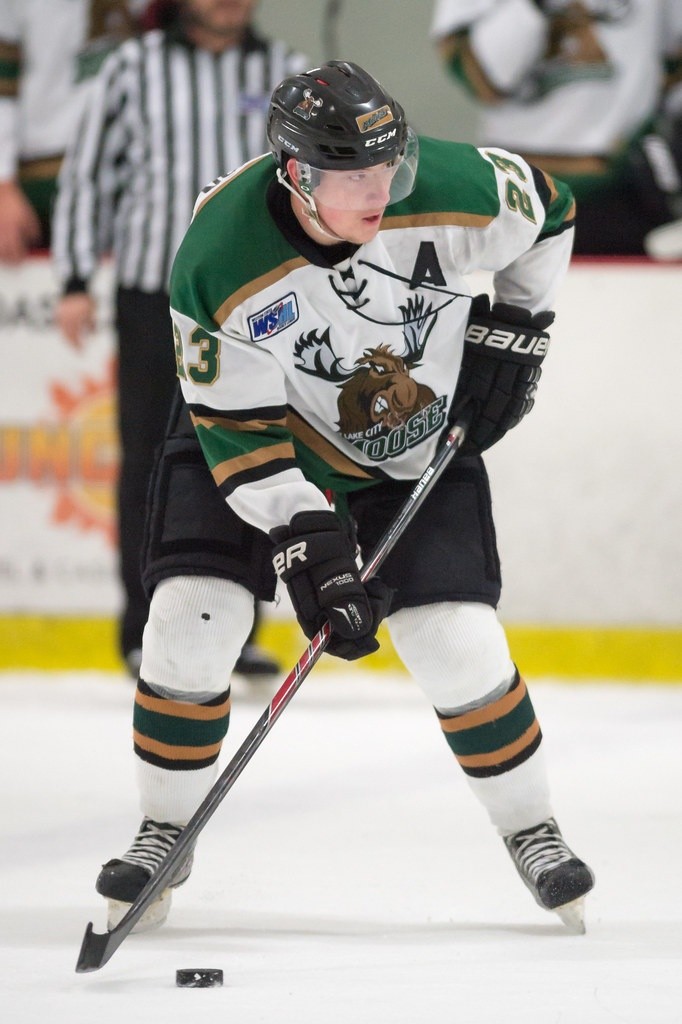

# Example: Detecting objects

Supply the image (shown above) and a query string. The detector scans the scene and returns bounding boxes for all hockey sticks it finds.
[75,409,470,972]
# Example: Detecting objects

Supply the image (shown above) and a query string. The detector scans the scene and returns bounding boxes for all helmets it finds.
[266,60,408,191]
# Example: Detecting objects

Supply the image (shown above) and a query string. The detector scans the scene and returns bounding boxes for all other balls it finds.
[172,969,223,987]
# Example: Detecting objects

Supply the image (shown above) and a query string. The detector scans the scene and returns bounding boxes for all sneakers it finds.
[124,648,143,678]
[96,816,196,935]
[232,645,282,701]
[503,817,594,933]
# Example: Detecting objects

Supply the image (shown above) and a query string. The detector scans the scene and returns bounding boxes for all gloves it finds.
[268,509,394,661]
[435,294,556,457]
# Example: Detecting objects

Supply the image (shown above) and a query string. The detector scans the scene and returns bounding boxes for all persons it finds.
[0,0,156,263]
[51,0,309,691]
[430,1,665,257]
[93,61,596,935]
